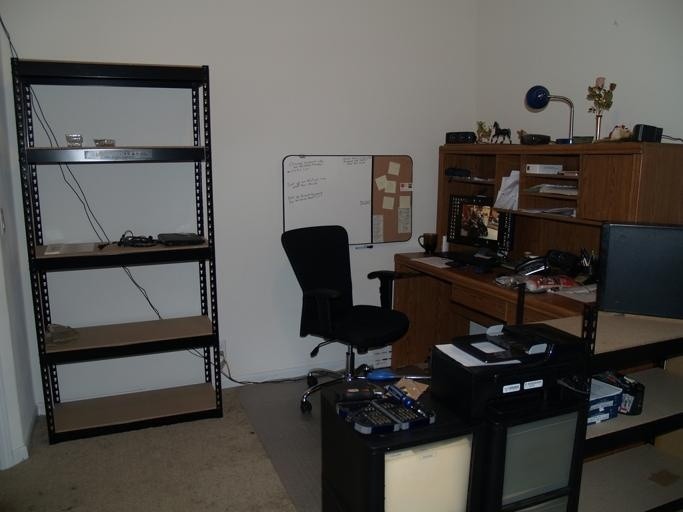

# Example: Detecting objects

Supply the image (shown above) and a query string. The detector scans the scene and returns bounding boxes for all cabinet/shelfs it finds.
[557,310,682,511]
[7,56,222,446]
[384,252,587,377]
[437,143,682,262]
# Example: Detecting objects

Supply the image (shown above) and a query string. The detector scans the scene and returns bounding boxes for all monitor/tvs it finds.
[446,193,516,267]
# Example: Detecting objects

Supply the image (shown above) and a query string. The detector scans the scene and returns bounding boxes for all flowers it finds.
[584,76,618,114]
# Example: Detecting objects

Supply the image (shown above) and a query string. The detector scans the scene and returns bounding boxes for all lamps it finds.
[525,86,577,146]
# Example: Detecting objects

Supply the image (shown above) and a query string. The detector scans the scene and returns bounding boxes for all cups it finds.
[418,232,438,257]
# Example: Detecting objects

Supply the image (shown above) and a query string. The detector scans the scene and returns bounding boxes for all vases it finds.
[595,115,602,141]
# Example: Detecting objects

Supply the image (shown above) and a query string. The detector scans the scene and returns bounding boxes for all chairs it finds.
[279,224,411,415]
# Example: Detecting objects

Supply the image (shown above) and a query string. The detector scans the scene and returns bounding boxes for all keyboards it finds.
[416,256,454,269]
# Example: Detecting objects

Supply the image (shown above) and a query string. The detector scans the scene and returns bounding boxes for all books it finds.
[523,183,578,197]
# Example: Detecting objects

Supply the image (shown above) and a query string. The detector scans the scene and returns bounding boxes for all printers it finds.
[430,323,584,424]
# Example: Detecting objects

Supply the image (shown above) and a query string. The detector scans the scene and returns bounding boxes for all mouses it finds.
[470,262,488,274]
